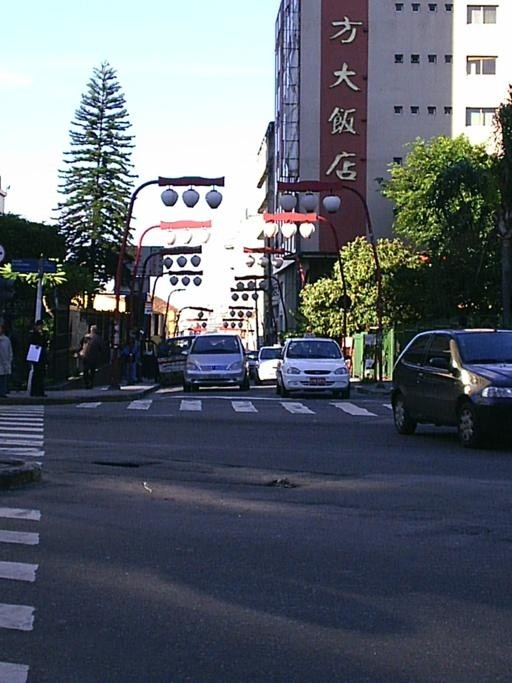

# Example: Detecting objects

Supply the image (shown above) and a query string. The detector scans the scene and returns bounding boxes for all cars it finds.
[389,326,512,448]
[277,337,352,398]
[254,344,284,386]
[246,351,259,378]
[184,334,251,392]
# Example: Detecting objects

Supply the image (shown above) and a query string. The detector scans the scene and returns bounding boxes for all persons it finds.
[124,329,143,387]
[0,327,16,396]
[81,323,99,392]
[24,317,51,399]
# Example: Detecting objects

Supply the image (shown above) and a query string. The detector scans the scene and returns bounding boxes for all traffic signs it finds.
[12,259,56,272]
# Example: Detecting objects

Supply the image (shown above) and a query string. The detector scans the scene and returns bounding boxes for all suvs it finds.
[157,336,195,383]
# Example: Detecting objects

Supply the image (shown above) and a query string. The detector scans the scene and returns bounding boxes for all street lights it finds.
[244,245,305,285]
[107,175,225,392]
[260,211,349,358]
[222,274,288,335]
[127,218,214,334]
[276,178,384,389]
